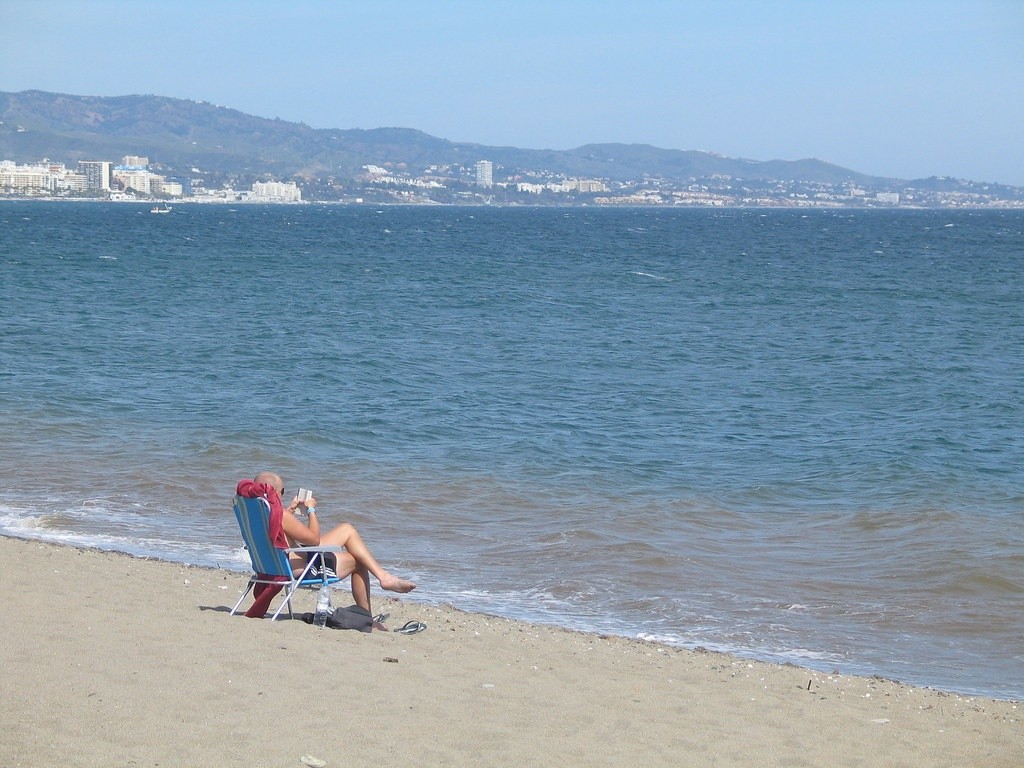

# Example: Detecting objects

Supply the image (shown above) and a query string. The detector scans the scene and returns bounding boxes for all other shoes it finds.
[372,614,390,623]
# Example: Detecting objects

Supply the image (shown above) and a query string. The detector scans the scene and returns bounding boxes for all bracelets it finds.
[306,508,318,516]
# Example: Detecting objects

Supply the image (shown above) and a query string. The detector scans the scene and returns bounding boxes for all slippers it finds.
[394,620,427,635]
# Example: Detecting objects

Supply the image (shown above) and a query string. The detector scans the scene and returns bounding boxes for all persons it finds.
[254,470,417,630]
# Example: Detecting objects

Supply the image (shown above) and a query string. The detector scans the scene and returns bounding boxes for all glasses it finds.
[276,488,284,496]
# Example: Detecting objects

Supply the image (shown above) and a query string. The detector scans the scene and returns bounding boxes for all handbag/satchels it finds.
[331,605,373,633]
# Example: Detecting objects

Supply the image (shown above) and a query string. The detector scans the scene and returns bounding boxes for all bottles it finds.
[312,582,330,626]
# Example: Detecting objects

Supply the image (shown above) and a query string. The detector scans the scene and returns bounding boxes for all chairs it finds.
[230,495,342,621]
